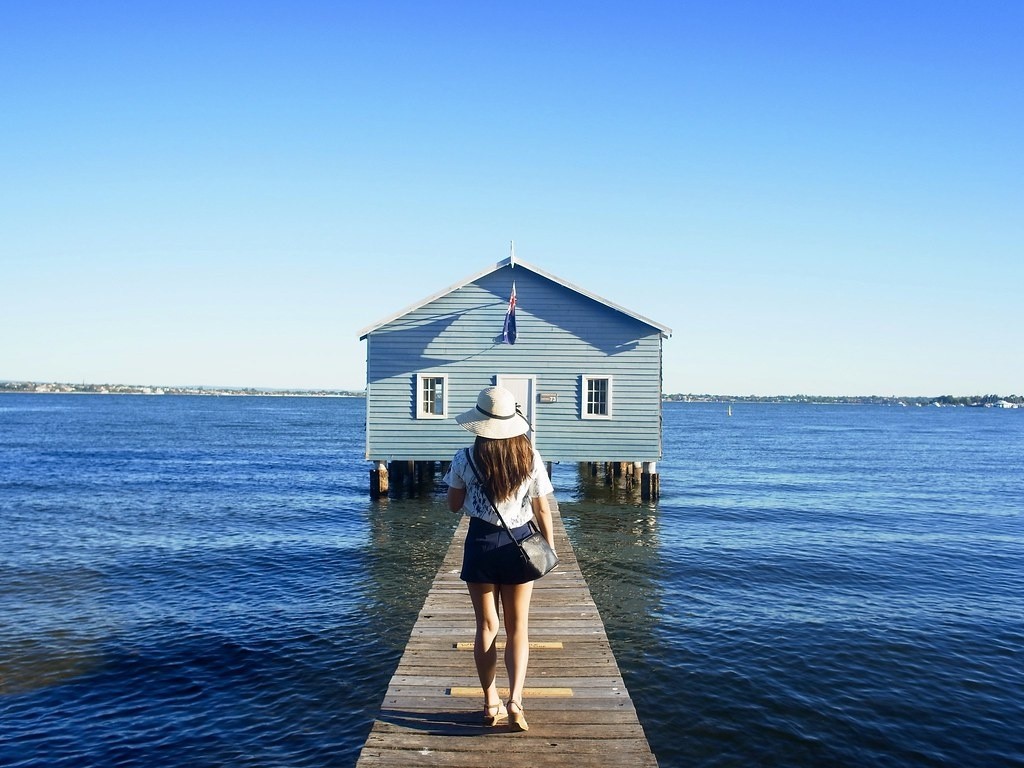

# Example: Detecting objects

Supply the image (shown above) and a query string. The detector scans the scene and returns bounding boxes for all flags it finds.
[502,288,517,345]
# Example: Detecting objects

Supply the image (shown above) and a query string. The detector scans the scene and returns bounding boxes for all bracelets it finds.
[551,548,556,552]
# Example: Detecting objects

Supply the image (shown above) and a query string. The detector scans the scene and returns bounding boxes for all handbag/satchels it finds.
[514,531,559,580]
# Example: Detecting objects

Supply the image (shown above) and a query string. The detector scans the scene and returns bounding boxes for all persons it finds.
[442,386,558,731]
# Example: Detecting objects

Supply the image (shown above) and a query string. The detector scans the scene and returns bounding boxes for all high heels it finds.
[506,700,528,731]
[482,699,505,727]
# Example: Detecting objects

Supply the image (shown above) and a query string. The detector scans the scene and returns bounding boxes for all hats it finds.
[455,387,530,439]
